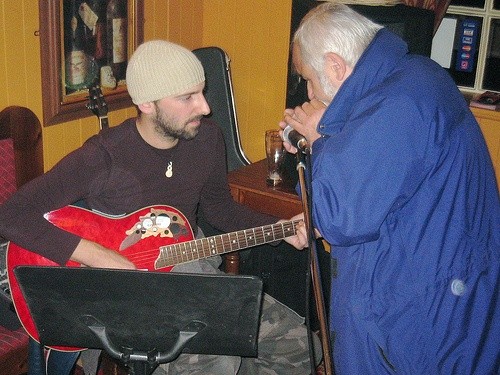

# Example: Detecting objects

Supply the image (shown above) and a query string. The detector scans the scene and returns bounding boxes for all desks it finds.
[223,155,310,275]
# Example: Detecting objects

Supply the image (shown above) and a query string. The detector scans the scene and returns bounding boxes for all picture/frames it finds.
[38,0,145,127]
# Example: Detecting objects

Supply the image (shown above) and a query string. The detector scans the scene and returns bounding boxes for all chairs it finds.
[190,46,253,172]
[0,104,60,374]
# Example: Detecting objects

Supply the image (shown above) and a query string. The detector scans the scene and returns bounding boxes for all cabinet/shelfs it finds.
[469,106,500,193]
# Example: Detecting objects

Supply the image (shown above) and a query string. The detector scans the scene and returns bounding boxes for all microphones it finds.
[282,124,308,152]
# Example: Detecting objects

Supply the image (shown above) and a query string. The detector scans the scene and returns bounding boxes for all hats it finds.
[126,40,205,104]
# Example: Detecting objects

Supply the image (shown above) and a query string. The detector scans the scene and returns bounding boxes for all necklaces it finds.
[138,128,181,178]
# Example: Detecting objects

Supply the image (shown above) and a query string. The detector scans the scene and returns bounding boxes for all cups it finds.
[264,129,292,187]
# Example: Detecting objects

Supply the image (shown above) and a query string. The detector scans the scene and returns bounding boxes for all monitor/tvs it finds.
[429,13,485,91]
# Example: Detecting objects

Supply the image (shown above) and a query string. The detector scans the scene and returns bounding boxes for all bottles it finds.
[104,0,126,79]
[87,1,106,69]
[64,0,90,86]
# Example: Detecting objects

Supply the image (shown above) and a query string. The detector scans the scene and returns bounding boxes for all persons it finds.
[275,1,500,375]
[1,41,323,374]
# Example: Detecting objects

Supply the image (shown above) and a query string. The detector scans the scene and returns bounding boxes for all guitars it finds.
[6,205,305,352]
[87,85,109,132]
